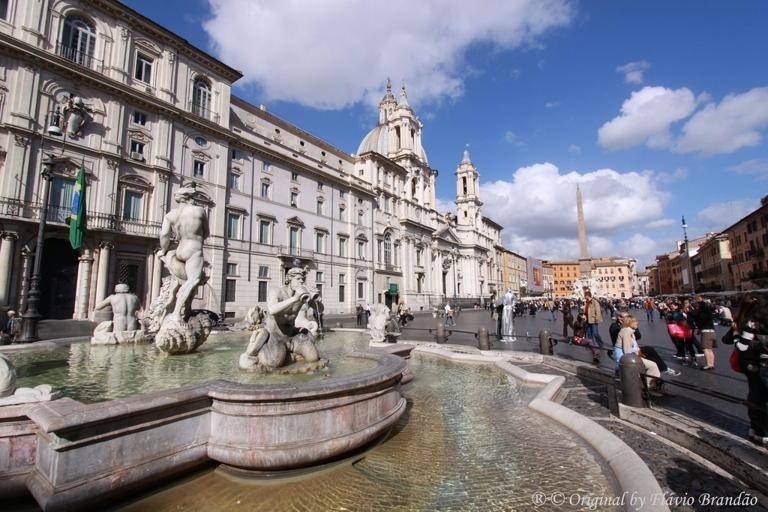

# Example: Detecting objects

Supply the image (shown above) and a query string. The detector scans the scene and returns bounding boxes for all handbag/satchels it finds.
[700,335,718,348]
[730,349,748,375]
[668,323,692,339]
[741,342,768,373]
[760,353,768,387]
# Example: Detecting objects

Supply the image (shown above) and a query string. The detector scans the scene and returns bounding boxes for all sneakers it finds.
[673,350,717,370]
[444,322,457,328]
[747,427,768,444]
[591,359,600,364]
[665,367,680,377]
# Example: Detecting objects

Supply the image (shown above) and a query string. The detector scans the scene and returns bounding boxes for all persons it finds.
[365,304,371,320]
[1,310,16,335]
[405,307,414,323]
[240,267,318,372]
[398,301,405,327]
[356,304,363,326]
[95,284,140,333]
[444,301,456,327]
[318,299,325,329]
[158,187,209,316]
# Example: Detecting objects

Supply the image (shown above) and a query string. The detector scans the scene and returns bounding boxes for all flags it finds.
[68,157,87,250]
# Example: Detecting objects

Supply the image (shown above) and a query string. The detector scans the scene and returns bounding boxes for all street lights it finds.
[517,265,520,304]
[15,105,68,344]
[658,268,663,301]
[681,215,696,295]
